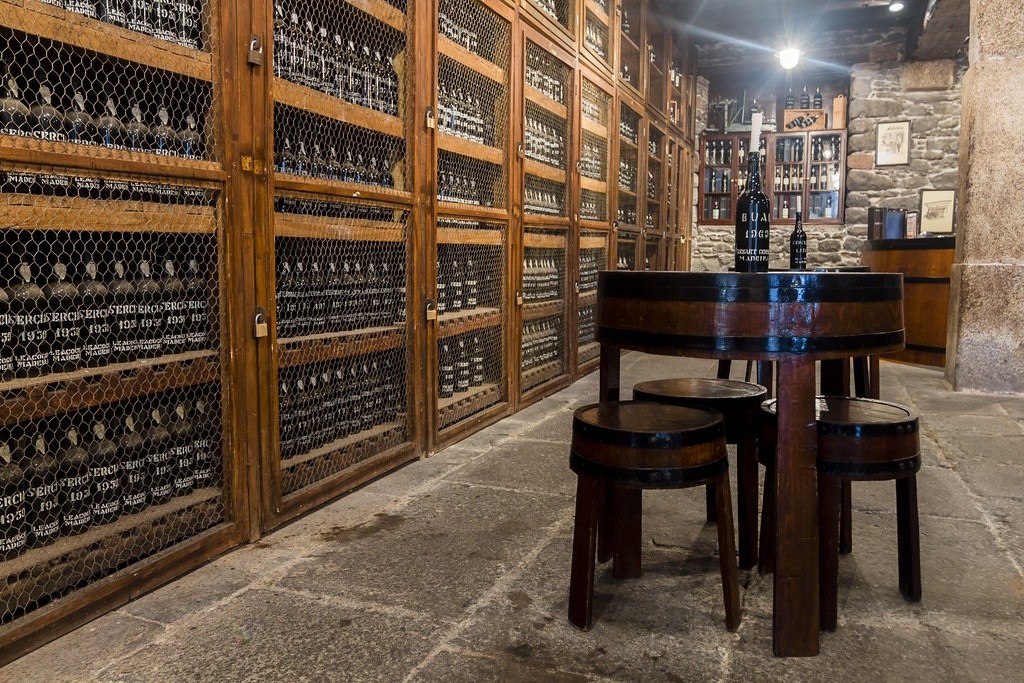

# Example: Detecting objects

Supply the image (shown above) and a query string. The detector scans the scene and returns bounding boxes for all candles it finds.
[749,111,763,151]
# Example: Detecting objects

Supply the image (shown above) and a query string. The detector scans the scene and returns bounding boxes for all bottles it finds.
[703,110,841,217]
[0,0,690,562]
[800,84,809,110]
[813,86,823,109]
[735,151,771,272]
[790,212,807,268]
[784,88,794,110]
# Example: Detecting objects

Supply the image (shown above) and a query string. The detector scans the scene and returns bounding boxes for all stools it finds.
[568,399,742,634]
[760,394,921,631]
[632,376,766,569]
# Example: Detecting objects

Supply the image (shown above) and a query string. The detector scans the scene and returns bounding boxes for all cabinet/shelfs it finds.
[701,129,848,225]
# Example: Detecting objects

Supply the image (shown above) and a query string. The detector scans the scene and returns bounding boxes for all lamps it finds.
[888,0,905,13]
[774,36,805,69]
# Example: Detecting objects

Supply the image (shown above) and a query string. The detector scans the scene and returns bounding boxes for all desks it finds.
[593,269,908,659]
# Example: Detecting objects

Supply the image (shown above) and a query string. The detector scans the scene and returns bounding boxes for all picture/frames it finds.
[875,120,911,166]
[917,188,957,234]
